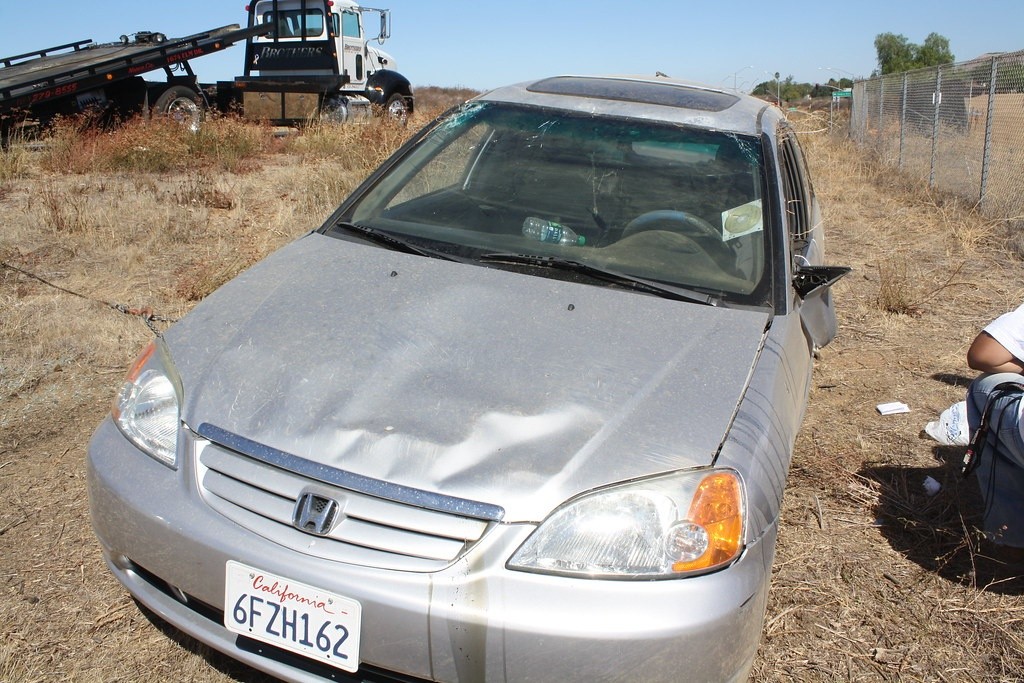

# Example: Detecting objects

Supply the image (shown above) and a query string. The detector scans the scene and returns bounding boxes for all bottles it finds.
[520,217,586,246]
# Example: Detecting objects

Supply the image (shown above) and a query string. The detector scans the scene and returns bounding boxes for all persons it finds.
[951,299,1024,570]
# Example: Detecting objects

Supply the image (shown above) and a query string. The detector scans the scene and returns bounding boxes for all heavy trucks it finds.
[2,0,417,148]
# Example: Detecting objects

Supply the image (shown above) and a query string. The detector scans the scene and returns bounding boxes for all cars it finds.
[88,70,851,683]
